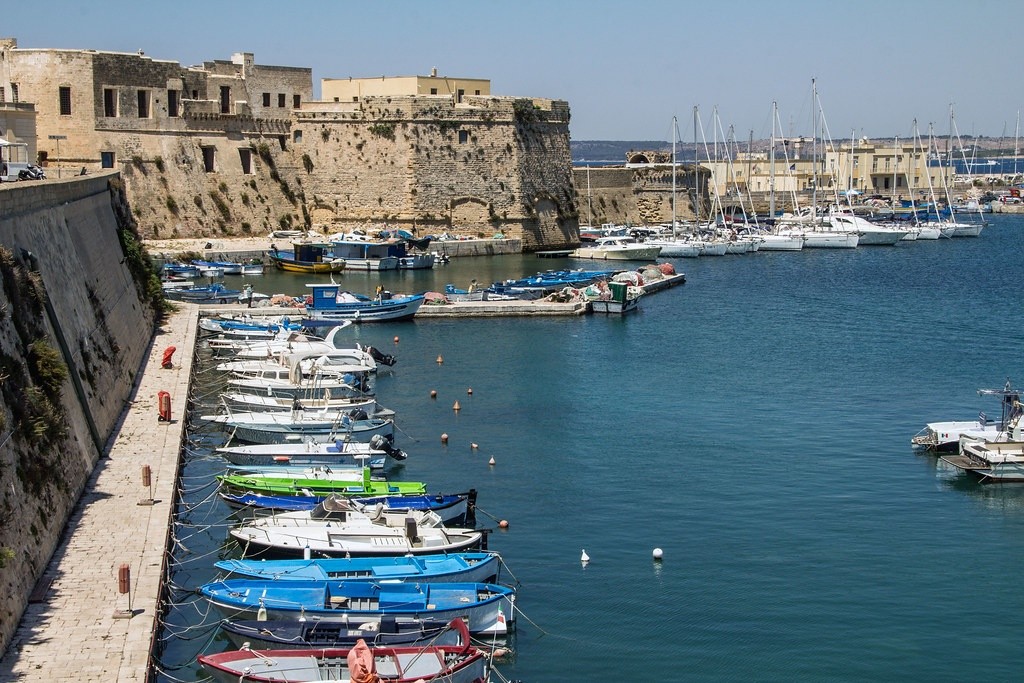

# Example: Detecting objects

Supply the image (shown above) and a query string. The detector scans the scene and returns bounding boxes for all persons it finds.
[81,167,85,175]
[247,284,254,308]
[3,165,7,176]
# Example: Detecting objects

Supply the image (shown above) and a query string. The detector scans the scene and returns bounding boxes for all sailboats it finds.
[569,76,1024,260]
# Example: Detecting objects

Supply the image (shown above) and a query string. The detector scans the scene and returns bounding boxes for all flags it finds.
[790,164,795,170]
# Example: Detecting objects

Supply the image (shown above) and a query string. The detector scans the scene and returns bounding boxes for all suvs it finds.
[999,194,1021,204]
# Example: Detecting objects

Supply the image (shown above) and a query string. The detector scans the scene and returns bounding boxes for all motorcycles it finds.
[18,161,48,181]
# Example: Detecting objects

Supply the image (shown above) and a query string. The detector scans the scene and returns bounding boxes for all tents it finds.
[846,189,858,195]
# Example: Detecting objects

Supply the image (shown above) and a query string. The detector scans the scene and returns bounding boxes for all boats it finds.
[196,313,492,560]
[195,580,519,637]
[910,376,1024,453]
[197,619,495,683]
[213,548,505,584]
[939,401,1024,484]
[444,266,628,301]
[305,284,426,322]
[302,291,372,303]
[266,228,450,273]
[220,614,470,652]
[161,253,273,304]
